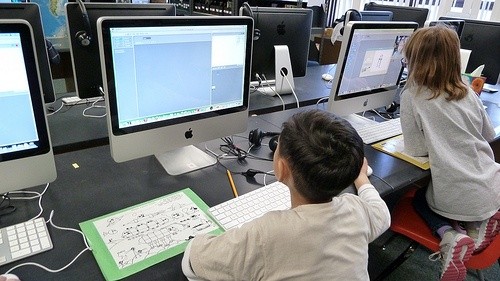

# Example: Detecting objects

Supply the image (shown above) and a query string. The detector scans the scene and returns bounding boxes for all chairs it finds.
[373,185,500,281]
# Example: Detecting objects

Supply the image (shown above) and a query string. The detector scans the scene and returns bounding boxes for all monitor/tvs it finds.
[0,2,56,104]
[0,18,56,215]
[342,2,500,92]
[97,11,254,177]
[239,6,313,96]
[63,2,178,99]
[324,19,419,128]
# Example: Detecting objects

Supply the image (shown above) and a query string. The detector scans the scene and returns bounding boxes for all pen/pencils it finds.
[227,169,238,198]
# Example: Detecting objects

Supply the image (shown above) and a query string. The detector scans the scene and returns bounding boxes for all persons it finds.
[399,25,500,281]
[190,109,391,281]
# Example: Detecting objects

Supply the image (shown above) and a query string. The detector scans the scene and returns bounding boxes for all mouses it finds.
[322,73,334,80]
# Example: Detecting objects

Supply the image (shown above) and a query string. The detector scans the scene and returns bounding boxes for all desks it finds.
[0,36,499,281]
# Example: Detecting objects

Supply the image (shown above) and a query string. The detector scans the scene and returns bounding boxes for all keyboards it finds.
[209,180,292,232]
[356,116,404,145]
[0,217,54,267]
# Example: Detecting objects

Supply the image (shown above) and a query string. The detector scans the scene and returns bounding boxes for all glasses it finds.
[400,57,410,66]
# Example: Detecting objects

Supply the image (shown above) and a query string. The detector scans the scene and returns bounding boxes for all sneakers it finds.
[456,210,500,256]
[428,228,475,281]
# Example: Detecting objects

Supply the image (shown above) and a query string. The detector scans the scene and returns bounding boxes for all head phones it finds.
[248,128,281,151]
[75,0,92,47]
[243,2,261,40]
[339,9,362,36]
